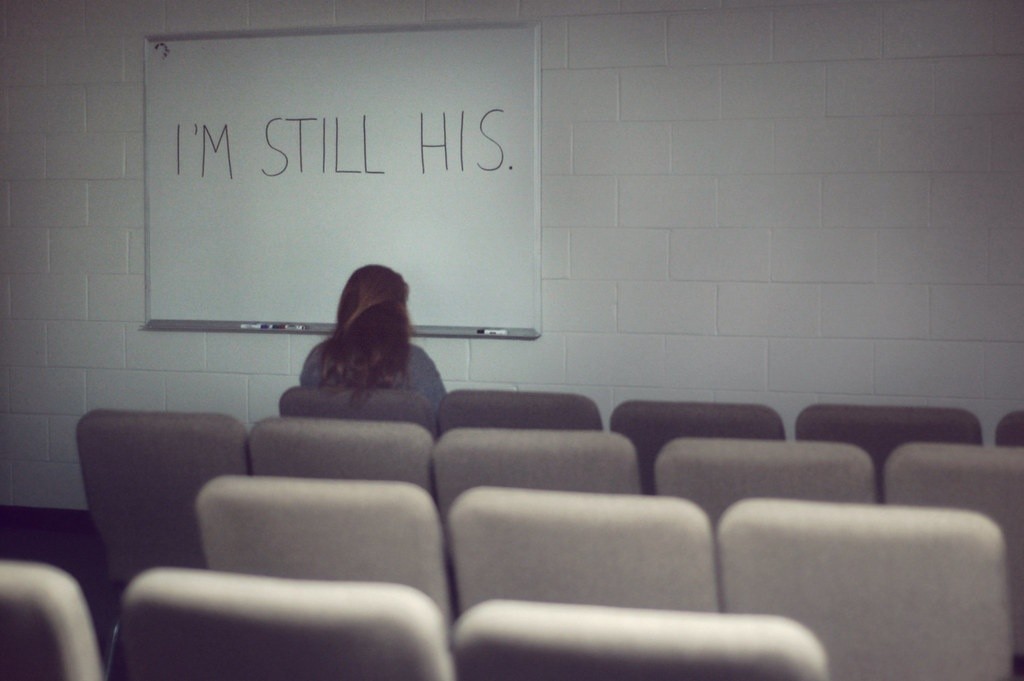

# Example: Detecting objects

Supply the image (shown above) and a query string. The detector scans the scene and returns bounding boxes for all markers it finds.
[241,323,305,330]
[477,328,508,336]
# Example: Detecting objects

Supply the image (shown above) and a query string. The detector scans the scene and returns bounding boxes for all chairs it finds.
[0,388,1024,681]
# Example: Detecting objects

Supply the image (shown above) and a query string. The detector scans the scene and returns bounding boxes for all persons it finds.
[299,265,446,415]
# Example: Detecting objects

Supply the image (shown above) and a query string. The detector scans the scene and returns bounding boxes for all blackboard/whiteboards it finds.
[137,28,546,338]
